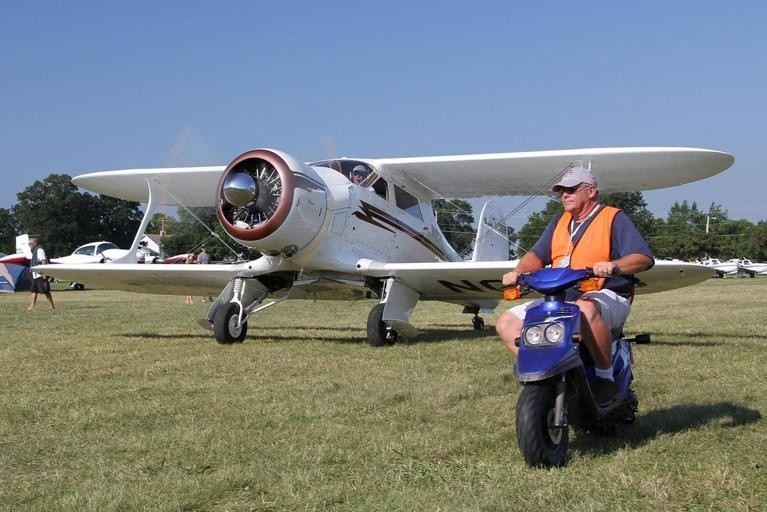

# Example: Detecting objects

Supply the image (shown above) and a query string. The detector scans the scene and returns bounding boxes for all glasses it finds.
[557,184,588,195]
[353,172,364,176]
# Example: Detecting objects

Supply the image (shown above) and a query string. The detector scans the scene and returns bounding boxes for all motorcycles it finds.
[498,267,650,468]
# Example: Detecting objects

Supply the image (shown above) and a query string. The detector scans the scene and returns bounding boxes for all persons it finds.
[195,243,214,303]
[184,252,197,305]
[349,165,377,194]
[491,165,658,409]
[27,237,56,312]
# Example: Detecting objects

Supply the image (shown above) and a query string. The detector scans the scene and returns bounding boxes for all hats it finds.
[201,243,207,249]
[552,166,598,195]
[352,165,369,173]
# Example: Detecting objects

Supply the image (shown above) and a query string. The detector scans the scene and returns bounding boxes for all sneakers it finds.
[201,297,213,304]
[594,375,620,409]
[186,301,194,305]
